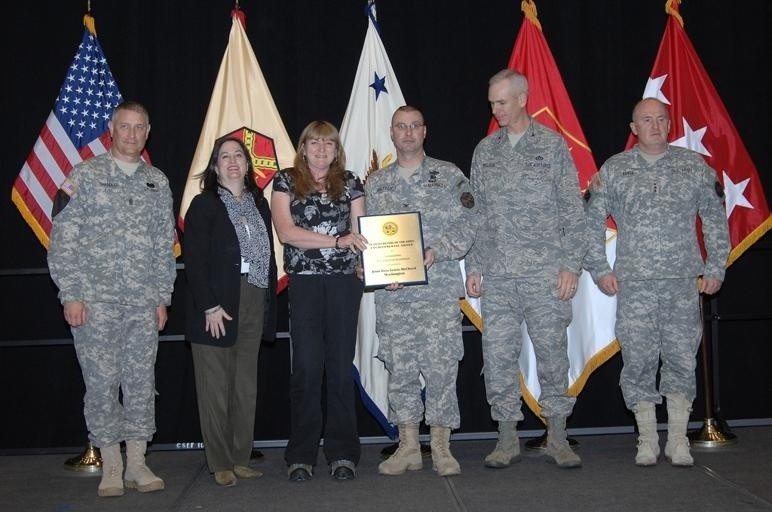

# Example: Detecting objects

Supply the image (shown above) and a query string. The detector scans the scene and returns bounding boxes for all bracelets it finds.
[335,237,340,249]
[205,305,221,315]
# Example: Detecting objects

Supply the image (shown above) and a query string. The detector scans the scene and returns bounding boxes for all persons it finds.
[270,120,369,482]
[47,103,179,498]
[464,69,589,468]
[180,136,278,488]
[583,98,728,466]
[362,105,477,477]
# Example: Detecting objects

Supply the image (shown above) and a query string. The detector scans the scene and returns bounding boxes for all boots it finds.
[543,416,581,468]
[430,428,461,476]
[665,391,693,466]
[98,444,125,496]
[378,423,423,476]
[125,440,165,493]
[485,421,521,468]
[633,402,661,466]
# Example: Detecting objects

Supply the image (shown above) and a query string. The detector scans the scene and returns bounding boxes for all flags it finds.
[606,1,771,292]
[177,11,298,296]
[339,1,464,439]
[459,0,622,427]
[11,15,182,258]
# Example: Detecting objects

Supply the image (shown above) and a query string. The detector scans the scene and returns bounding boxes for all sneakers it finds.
[286,462,312,481]
[329,460,355,479]
[214,470,238,487]
[234,465,263,479]
